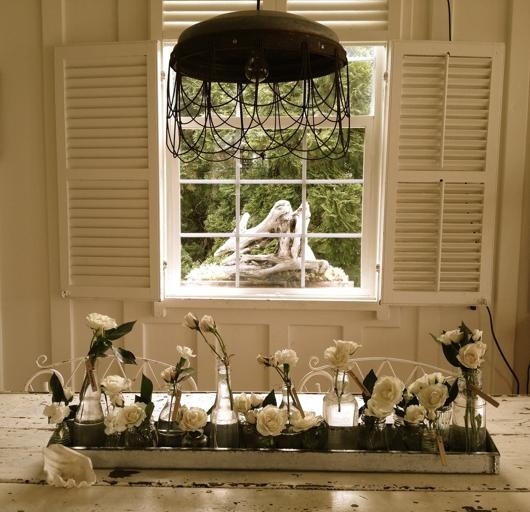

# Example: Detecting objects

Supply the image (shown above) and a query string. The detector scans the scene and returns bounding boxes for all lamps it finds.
[162,0,352,164]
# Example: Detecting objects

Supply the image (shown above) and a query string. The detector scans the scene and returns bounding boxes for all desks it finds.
[1,385,529,511]
